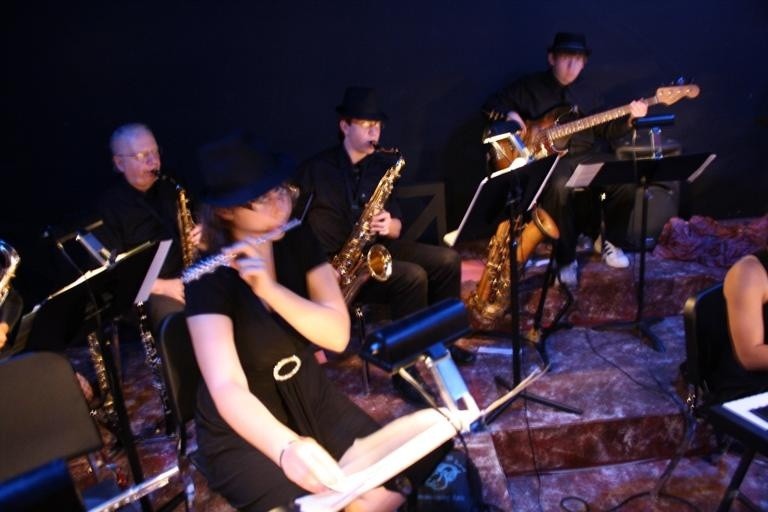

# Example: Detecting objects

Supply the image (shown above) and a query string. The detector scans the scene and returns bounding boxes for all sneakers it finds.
[601,240,630,268]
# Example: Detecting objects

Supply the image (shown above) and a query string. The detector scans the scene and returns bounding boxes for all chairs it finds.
[653,284,740,499]
[0,350,135,512]
[154,312,234,512]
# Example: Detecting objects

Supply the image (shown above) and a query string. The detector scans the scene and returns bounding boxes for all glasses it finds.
[346,118,386,130]
[239,182,300,212]
[115,148,164,161]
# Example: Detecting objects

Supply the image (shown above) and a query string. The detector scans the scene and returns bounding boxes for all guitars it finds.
[484,85,701,168]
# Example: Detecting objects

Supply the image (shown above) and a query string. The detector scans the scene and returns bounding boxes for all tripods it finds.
[444,151,583,421]
[16,240,186,512]
[469,202,576,372]
[591,153,702,355]
[293,365,547,512]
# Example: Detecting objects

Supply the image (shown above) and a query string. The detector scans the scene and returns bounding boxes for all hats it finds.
[196,144,297,207]
[547,32,590,52]
[336,87,389,121]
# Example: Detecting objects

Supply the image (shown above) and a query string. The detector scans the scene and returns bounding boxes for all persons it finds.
[482,30,637,291]
[88,122,186,328]
[709,247,768,436]
[177,145,416,512]
[293,89,476,405]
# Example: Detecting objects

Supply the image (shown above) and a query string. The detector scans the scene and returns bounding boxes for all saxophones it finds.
[466,208,560,331]
[329,142,406,309]
[149,168,197,270]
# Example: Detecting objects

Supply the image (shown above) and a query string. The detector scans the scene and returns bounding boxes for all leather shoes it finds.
[449,345,476,365]
[391,364,437,407]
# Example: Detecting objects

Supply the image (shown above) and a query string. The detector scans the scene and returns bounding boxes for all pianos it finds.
[696,383,768,458]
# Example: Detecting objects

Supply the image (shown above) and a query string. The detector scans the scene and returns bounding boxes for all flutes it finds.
[180,218,303,285]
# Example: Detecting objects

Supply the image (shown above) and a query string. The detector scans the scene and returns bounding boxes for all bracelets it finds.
[279,441,294,467]
